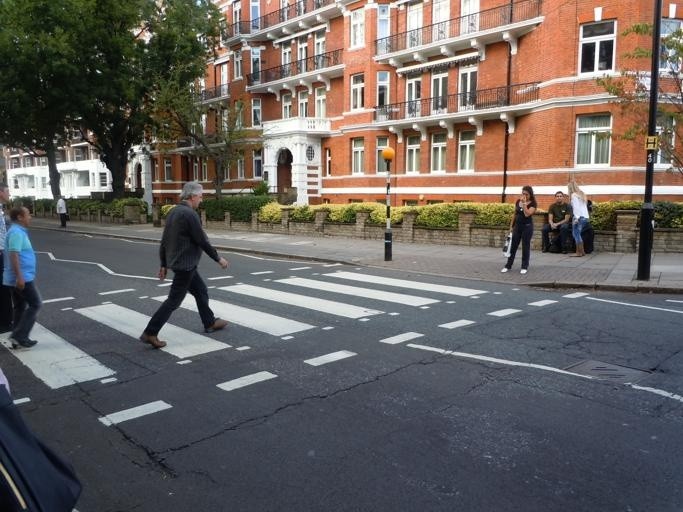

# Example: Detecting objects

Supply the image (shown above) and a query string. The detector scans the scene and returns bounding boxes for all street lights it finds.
[380,146,396,262]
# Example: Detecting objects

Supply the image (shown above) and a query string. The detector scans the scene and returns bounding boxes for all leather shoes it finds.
[9,335,39,351]
[204,317,228,333]
[139,333,168,348]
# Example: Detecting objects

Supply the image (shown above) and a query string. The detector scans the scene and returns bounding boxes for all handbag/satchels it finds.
[587,199,593,213]
[503,230,512,257]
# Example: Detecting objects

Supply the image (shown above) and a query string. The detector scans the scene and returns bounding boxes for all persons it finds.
[2,206,42,350]
[138,180,230,349]
[541,180,594,257]
[0,182,14,335]
[500,185,537,276]
[54,194,67,229]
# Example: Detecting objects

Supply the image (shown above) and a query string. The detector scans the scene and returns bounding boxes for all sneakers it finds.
[501,267,511,273]
[520,268,527,275]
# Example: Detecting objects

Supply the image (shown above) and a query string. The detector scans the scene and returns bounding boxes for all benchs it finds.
[541,212,595,253]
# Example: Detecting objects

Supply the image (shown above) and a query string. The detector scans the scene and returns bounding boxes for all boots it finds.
[570,243,585,257]
